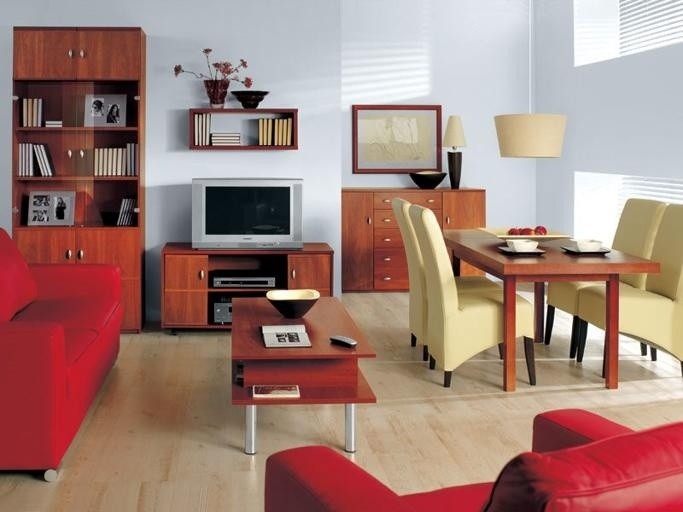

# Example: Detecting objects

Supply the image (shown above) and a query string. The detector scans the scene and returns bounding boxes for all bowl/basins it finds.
[231,91,269,109]
[567,239,602,252]
[408,171,447,190]
[506,240,537,251]
[265,289,319,317]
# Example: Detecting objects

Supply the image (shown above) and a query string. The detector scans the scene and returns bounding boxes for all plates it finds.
[476,227,575,242]
[497,251,545,260]
[560,246,611,255]
[496,246,546,255]
[560,251,611,262]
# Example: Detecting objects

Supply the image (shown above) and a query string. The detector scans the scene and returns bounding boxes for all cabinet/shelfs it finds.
[160,242,334,335]
[13,26,145,336]
[187,108,299,150]
[341,187,485,293]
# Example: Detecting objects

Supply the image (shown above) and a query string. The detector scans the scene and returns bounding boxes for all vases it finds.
[203,79,230,108]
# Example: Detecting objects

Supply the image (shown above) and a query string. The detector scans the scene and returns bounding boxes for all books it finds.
[260,322,312,348]
[94,141,138,226]
[16,97,63,178]
[194,113,241,146]
[258,118,293,146]
[252,384,301,400]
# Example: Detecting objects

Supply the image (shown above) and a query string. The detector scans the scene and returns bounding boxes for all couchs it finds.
[0,226,118,485]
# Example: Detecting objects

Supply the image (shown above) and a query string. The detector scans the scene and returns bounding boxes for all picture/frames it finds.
[26,185,78,227]
[82,90,128,128]
[351,104,441,174]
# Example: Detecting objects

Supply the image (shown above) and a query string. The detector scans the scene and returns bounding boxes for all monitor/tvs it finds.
[191,177,304,249]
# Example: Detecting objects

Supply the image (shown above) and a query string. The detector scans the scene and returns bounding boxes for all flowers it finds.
[173,46,252,88]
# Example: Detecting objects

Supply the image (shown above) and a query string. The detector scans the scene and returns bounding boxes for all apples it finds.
[509,226,547,235]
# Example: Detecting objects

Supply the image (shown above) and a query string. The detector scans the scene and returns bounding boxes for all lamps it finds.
[441,114,465,188]
[489,0,570,161]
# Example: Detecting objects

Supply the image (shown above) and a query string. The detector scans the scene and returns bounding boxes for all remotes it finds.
[330,335,358,347]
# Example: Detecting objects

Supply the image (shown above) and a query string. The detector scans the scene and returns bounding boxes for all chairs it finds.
[408,202,537,387]
[262,409,681,512]
[390,198,501,360]
[576,204,683,367]
[544,198,668,359]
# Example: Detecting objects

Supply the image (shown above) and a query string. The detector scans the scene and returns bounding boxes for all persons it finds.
[32,195,66,222]
[107,104,121,123]
[91,100,105,118]
